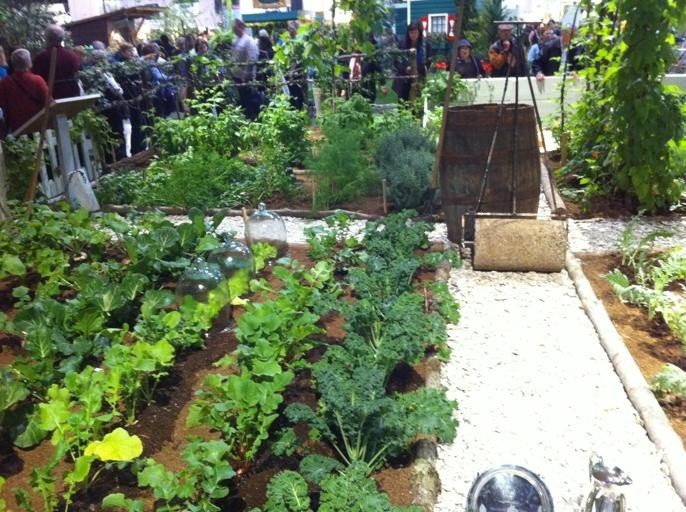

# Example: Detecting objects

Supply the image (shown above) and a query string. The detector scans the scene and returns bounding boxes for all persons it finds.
[668,37,686,73]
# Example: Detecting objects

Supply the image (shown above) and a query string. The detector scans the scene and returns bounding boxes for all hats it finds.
[457,39,473,49]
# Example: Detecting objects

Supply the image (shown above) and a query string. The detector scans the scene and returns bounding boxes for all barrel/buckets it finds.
[441,102,542,248]
